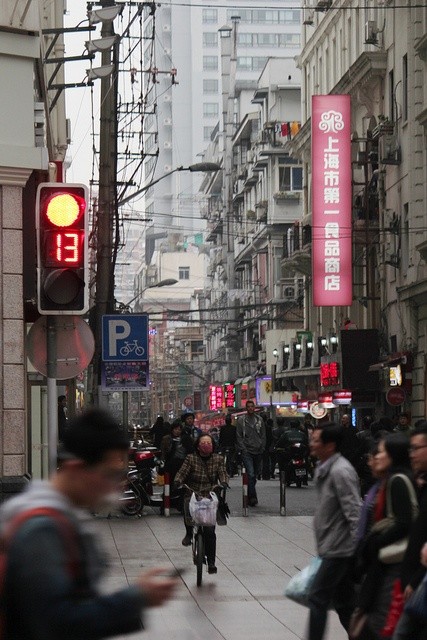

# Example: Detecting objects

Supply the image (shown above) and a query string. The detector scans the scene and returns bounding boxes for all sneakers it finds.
[183,534,193,545]
[208,564,216,572]
[248,497,257,505]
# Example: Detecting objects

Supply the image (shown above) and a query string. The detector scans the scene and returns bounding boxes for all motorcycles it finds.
[120,447,185,515]
[286,442,307,488]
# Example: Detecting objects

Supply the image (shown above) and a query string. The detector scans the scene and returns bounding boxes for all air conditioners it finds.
[272,284,296,300]
[364,21,377,44]
[378,135,395,161]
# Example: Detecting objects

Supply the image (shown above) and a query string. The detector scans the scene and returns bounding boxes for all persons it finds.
[340,414,427,476]
[266,419,275,480]
[0,407,178,640]
[256,415,267,480]
[278,420,309,488]
[306,423,364,640]
[236,401,266,506]
[348,446,381,640]
[160,421,170,441]
[388,427,426,640]
[150,417,163,433]
[162,424,195,479]
[58,395,67,438]
[218,414,238,478]
[272,418,291,442]
[181,413,203,451]
[351,433,419,640]
[174,433,229,574]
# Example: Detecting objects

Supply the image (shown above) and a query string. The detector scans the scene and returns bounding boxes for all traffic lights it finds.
[35,183,89,316]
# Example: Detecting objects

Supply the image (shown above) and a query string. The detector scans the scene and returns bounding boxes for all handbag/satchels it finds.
[215,496,230,526]
[370,518,409,564]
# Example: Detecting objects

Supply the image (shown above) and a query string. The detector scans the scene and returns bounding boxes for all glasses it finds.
[409,443,426,450]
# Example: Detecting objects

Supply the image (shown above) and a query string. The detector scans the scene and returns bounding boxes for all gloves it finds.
[221,481,227,489]
[171,481,181,492]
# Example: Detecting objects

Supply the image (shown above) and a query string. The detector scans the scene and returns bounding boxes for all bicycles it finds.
[222,448,233,477]
[177,482,231,587]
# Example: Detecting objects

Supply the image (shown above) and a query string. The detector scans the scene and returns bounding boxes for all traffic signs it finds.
[102,314,148,362]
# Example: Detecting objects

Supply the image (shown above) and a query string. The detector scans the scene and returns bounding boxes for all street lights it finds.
[92,163,221,385]
[122,279,178,432]
